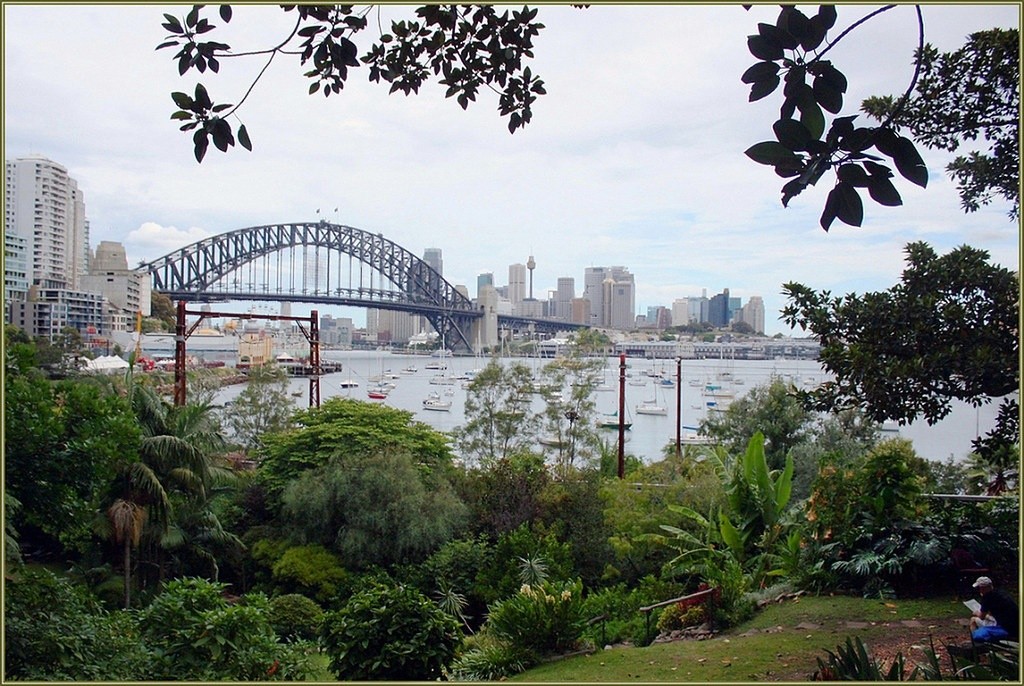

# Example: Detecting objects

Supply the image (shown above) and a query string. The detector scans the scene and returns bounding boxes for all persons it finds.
[970,577,1019,642]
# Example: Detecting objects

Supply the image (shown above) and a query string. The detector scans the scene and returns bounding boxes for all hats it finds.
[972,576,992,587]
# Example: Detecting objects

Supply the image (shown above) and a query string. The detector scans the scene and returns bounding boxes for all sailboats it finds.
[595,369,634,430]
[527,327,744,412]
[634,353,668,416]
[341,325,484,412]
[668,385,720,446]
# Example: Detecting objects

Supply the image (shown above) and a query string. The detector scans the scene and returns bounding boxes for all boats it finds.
[538,435,569,447]
[786,350,821,388]
[877,419,899,431]
